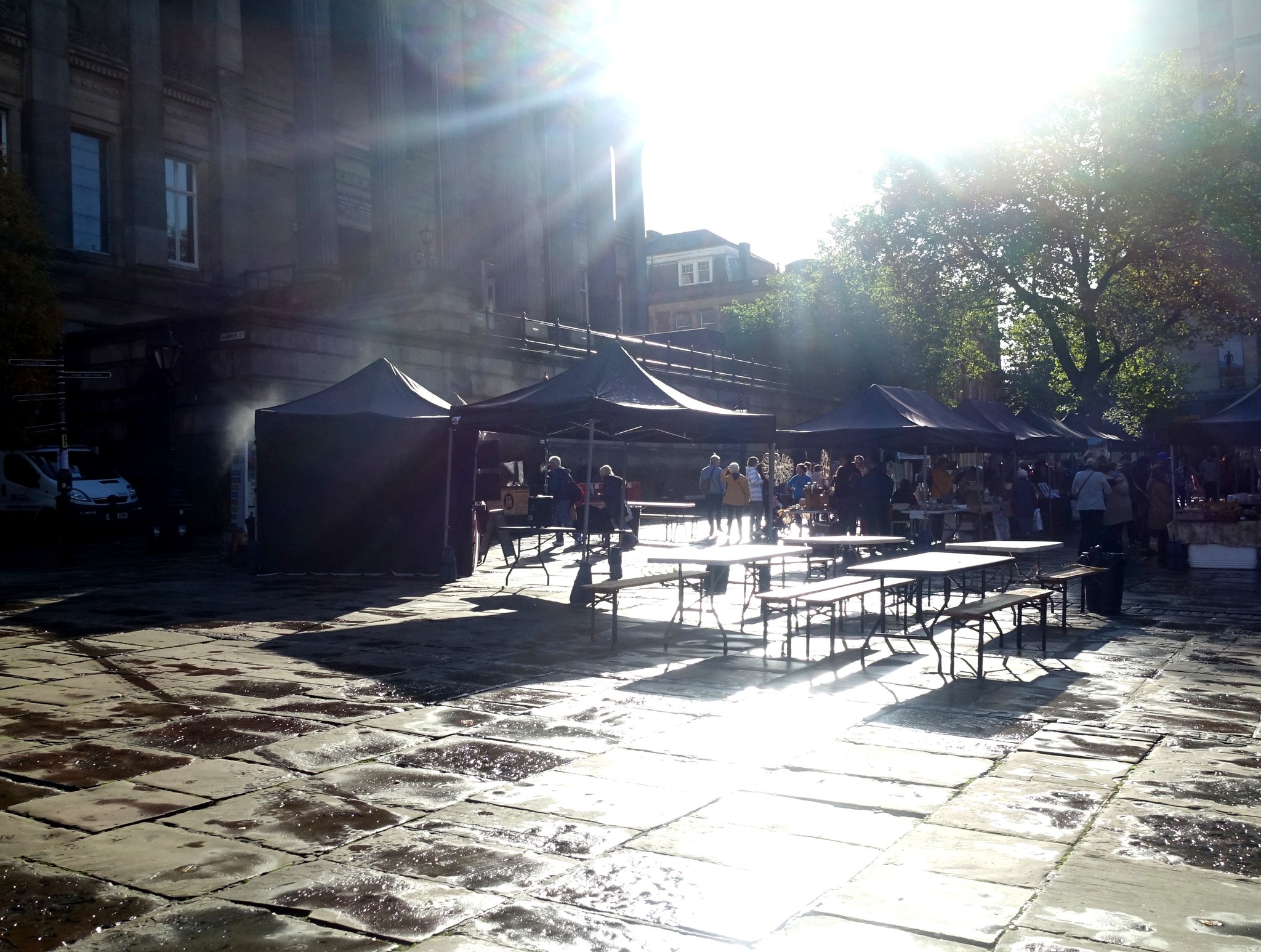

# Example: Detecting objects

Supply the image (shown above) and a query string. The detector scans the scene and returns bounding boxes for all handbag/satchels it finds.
[569,473,584,522]
[702,480,710,493]
[940,493,952,504]
[1071,500,1081,521]
[1031,508,1044,535]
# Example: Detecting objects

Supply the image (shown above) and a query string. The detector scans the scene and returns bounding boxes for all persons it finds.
[700,454,726,536]
[578,457,598,483]
[597,465,639,549]
[721,462,750,546]
[745,452,1260,563]
[547,455,583,547]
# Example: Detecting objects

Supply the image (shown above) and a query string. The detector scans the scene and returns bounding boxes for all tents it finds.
[439,338,777,576]
[778,374,1261,540]
[251,357,481,579]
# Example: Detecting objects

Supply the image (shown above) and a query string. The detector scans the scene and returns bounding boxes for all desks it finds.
[782,537,905,610]
[902,510,958,545]
[626,502,696,542]
[943,540,1063,626]
[578,499,641,540]
[957,501,1010,541]
[1168,519,1261,571]
[497,526,576,586]
[848,554,1016,674]
[646,545,811,657]
[786,511,837,535]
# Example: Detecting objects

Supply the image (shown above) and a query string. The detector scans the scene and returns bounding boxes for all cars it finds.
[2,443,137,523]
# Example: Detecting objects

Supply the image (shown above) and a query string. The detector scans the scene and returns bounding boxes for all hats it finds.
[1157,452,1172,462]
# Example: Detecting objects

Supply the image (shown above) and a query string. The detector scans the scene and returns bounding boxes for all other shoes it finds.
[600,542,611,547]
[552,540,564,545]
[749,530,766,537]
[631,540,639,548]
[1141,549,1159,556]
[573,537,583,548]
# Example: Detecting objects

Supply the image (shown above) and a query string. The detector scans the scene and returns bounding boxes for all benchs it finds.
[579,514,1106,680]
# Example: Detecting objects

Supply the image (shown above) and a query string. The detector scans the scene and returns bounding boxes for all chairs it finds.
[890,503,914,540]
